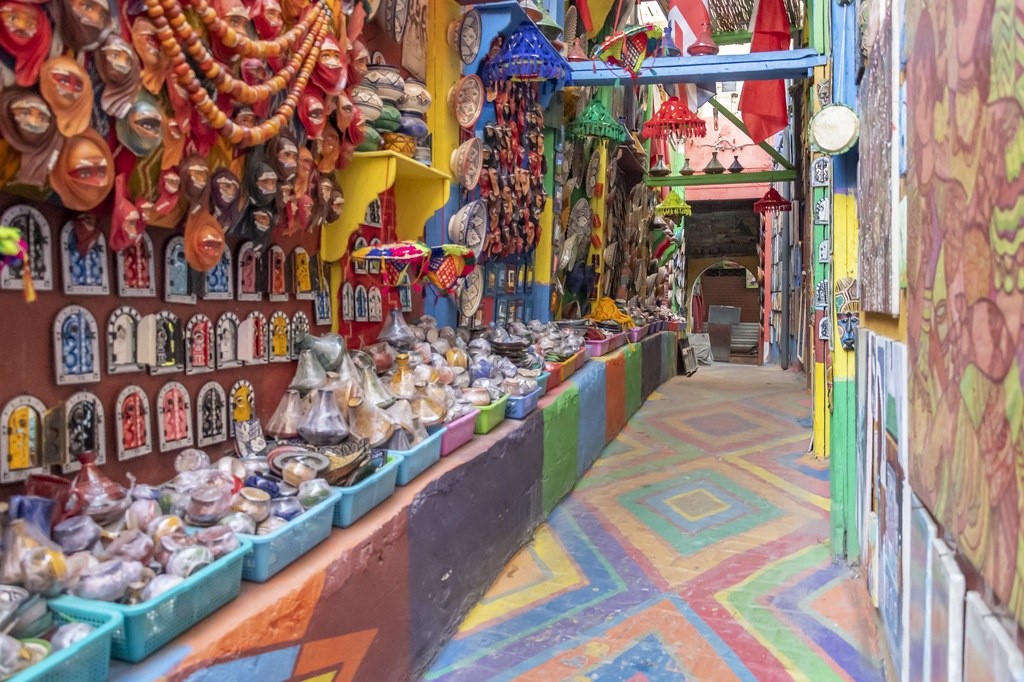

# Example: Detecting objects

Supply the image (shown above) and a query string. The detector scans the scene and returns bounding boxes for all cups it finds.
[11,473,84,541]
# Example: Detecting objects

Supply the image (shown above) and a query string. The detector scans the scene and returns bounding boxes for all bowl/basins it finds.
[446,265,483,317]
[449,137,483,190]
[447,10,483,65]
[446,74,484,128]
[376,0,409,44]
[602,181,644,294]
[550,319,588,336]
[448,199,486,260]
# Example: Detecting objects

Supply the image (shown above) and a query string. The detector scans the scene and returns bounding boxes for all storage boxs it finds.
[0,321,687,681]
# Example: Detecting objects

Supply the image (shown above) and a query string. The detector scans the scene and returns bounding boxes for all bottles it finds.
[264,307,447,448]
[727,156,744,173]
[657,27,682,56]
[567,38,590,62]
[350,64,433,165]
[648,155,672,177]
[619,116,648,168]
[687,22,719,56]
[489,321,511,343]
[679,159,695,176]
[519,0,564,53]
[702,152,726,174]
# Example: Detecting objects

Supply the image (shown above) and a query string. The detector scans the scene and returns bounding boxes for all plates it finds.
[489,335,534,364]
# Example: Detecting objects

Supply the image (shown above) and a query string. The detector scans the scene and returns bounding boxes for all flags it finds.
[737,0,791,144]
[659,0,716,114]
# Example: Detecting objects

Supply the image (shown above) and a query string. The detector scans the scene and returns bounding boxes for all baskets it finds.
[441,410,481,457]
[7,597,125,682]
[545,321,687,392]
[536,372,551,398]
[325,452,404,529]
[385,425,448,486]
[61,528,256,663]
[230,492,342,583]
[505,386,542,419]
[475,395,509,434]
